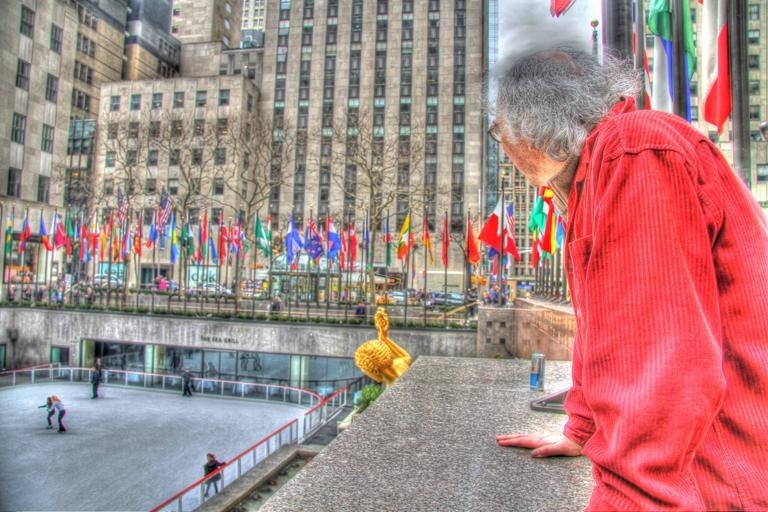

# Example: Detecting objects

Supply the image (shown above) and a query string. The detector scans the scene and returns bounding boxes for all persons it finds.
[203,351,263,380]
[488,33,768,512]
[48,394,68,433]
[354,306,412,389]
[203,452,225,497]
[6,274,513,325]
[169,350,180,370]
[120,354,127,370]
[38,396,56,430]
[89,357,104,402]
[181,371,193,398]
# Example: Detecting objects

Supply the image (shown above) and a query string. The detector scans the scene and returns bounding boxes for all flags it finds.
[620,0,733,137]
[5,186,369,270]
[398,216,414,259]
[465,218,481,266]
[442,218,450,267]
[422,217,433,264]
[527,186,570,268]
[384,221,392,267]
[478,194,519,272]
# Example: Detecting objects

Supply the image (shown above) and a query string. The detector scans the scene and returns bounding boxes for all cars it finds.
[203,282,232,294]
[385,288,464,306]
[91,274,124,288]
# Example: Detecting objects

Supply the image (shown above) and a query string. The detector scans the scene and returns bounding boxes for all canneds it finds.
[529,353,545,394]
